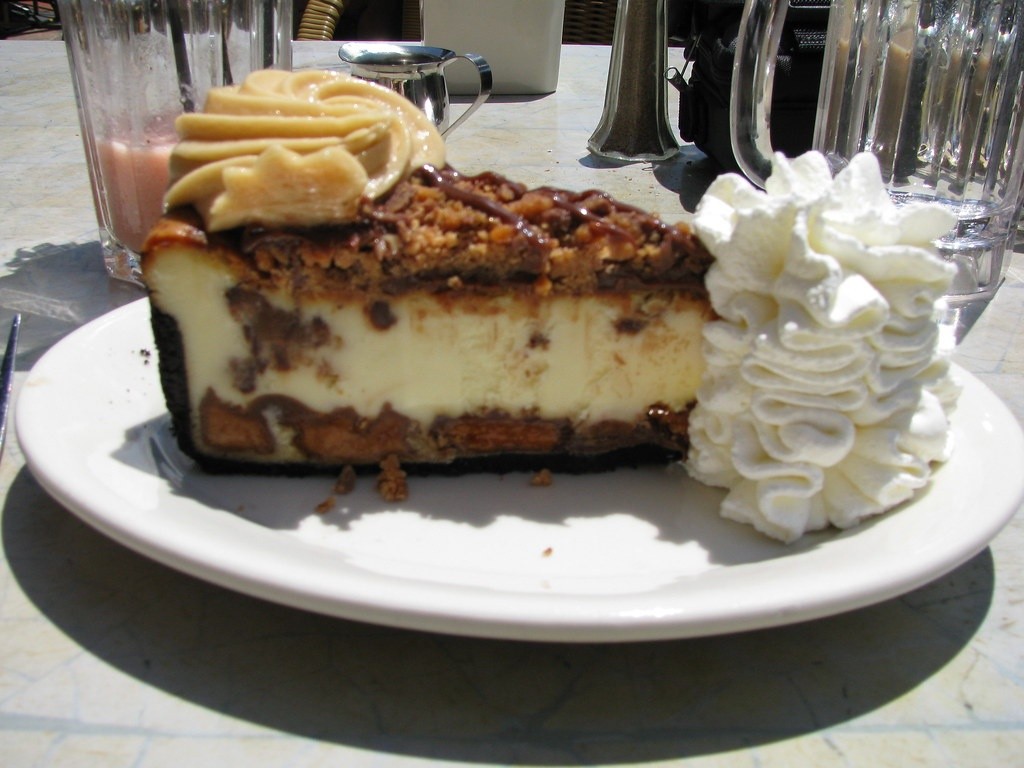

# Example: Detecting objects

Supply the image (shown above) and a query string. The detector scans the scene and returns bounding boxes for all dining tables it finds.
[0,39,1024,768]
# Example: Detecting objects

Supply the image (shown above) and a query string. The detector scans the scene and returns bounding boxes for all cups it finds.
[732,0,1024,347]
[50,0,295,288]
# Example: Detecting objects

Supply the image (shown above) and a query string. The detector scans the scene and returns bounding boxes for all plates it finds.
[14,297,1024,642]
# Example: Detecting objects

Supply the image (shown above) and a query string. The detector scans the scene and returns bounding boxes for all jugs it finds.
[338,43,492,143]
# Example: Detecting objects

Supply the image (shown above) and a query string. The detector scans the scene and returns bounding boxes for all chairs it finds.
[296,0,617,45]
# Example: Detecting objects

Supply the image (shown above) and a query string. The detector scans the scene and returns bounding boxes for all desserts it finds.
[132,68,961,544]
